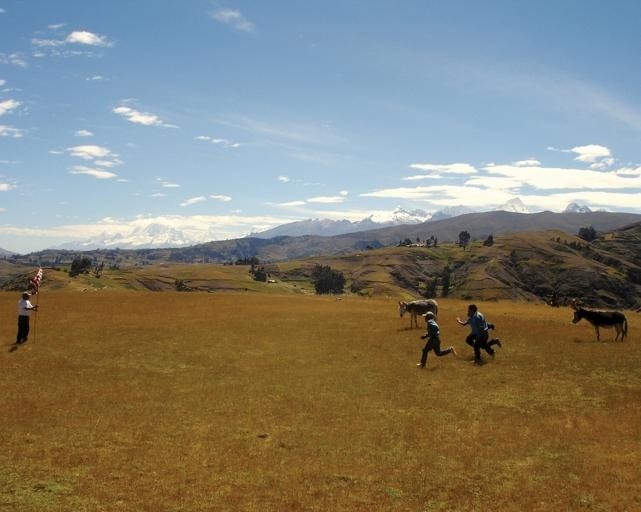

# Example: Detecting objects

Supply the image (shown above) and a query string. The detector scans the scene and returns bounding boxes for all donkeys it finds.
[572,306,627,343]
[398,299,438,329]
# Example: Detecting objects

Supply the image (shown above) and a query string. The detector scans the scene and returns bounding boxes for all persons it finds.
[467,304,496,365]
[415,311,458,369]
[456,310,502,356]
[15,291,40,345]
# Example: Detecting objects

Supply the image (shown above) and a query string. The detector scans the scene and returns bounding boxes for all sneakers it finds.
[450,346,457,356]
[495,338,501,348]
[416,364,426,368]
[492,351,496,360]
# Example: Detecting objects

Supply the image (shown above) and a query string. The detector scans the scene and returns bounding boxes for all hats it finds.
[23,292,31,297]
[422,312,433,319]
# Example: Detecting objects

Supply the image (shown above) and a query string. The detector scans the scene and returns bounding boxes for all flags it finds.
[30,267,43,296]
